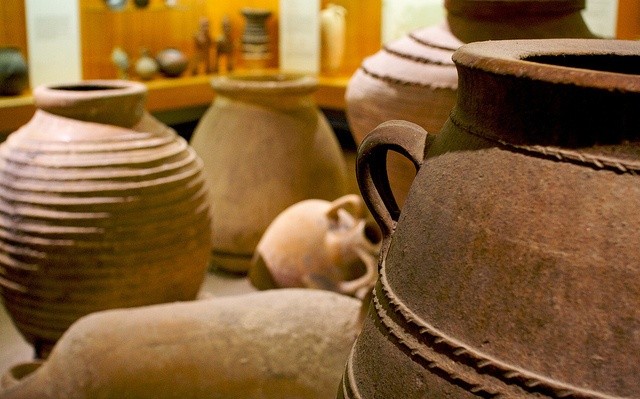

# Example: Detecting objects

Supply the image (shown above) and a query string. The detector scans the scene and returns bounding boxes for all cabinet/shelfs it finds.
[2,70,349,132]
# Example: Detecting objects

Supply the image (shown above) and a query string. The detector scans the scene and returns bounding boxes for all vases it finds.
[335,37,640,399]
[135,47,157,79]
[0,83,211,359]
[252,194,382,300]
[0,46,28,96]
[159,46,187,77]
[192,71,349,274]
[343,0,607,212]
[321,4,345,76]
[241,9,273,71]
[0,289,365,399]
